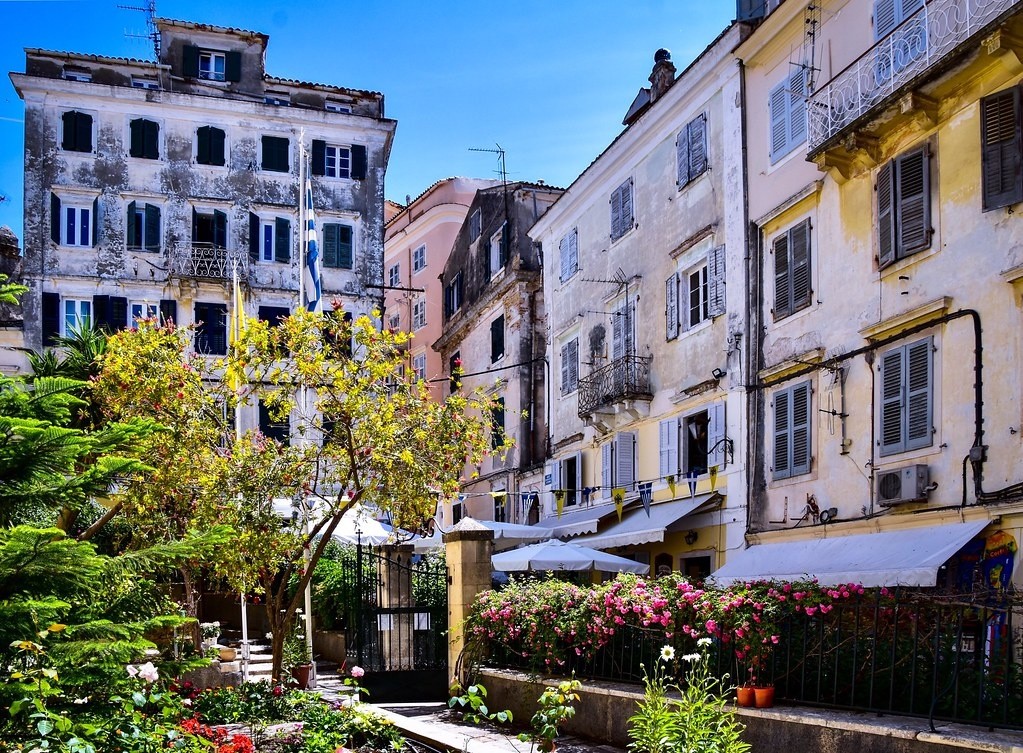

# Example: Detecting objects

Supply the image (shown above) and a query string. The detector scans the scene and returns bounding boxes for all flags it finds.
[221,285,249,392]
[303,165,321,312]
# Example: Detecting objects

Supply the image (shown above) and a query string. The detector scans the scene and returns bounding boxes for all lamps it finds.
[687,415,734,464]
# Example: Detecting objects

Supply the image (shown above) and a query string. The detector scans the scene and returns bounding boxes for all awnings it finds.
[567,492,718,550]
[533,498,637,539]
[704,519,994,591]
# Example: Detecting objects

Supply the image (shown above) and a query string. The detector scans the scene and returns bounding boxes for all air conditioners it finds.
[875,463,928,505]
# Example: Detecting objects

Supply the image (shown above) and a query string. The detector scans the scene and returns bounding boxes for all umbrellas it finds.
[280,510,420,550]
[407,517,552,555]
[489,537,649,574]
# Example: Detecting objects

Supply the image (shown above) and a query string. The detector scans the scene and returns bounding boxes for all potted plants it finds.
[282,663,313,691]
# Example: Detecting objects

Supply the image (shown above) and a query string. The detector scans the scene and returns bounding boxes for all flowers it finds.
[266,607,312,659]
[200,621,220,636]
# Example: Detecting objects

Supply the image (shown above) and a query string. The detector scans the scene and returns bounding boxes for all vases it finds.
[737,684,775,707]
[220,648,236,662]
[203,636,218,646]
